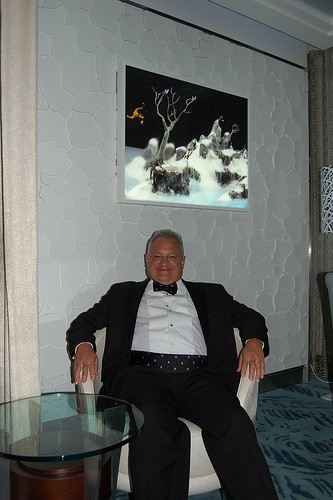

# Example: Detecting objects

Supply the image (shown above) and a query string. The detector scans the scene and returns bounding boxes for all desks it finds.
[0,392,144,500]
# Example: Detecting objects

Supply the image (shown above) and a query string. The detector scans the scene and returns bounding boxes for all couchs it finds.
[80,324,263,500]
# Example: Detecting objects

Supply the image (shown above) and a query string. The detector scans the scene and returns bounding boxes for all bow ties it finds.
[152,281,178,296]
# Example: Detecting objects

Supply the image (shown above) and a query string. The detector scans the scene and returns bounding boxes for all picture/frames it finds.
[118,64,249,212]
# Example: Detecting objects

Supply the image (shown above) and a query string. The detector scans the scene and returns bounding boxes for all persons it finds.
[66,229,279,500]
